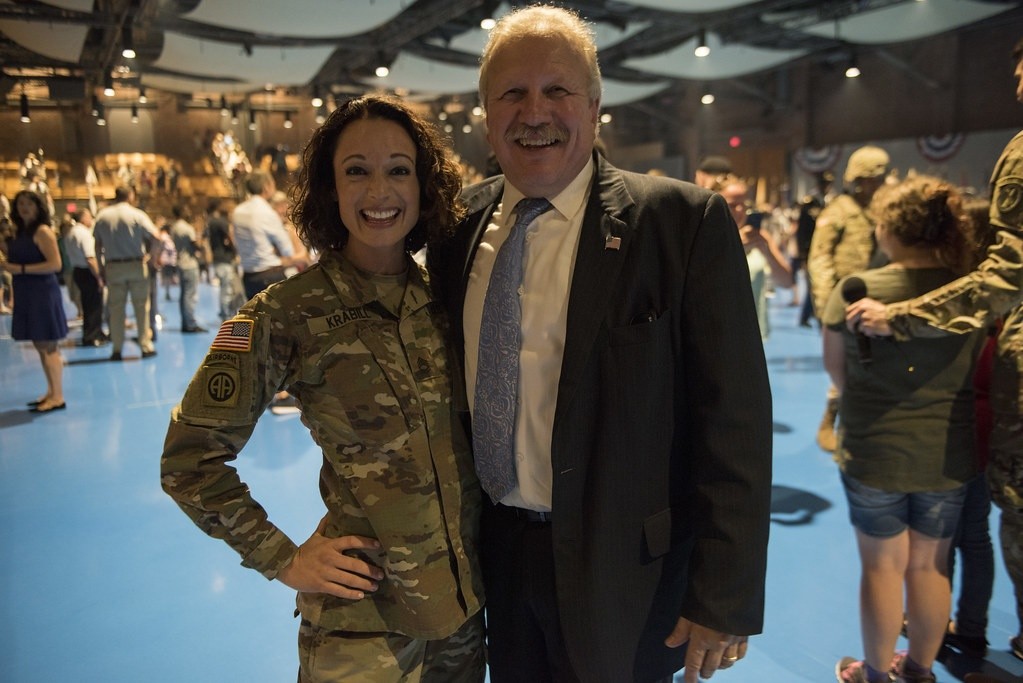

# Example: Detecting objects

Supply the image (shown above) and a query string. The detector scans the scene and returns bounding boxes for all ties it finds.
[473,196,556,506]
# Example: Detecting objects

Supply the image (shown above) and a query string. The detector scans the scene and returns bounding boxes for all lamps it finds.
[700,82,715,105]
[471,95,482,116]
[461,115,472,133]
[311,84,323,107]
[438,105,448,121]
[443,117,453,132]
[600,107,613,124]
[19,71,115,126]
[249,110,257,130]
[315,107,326,124]
[122,25,135,59]
[695,30,710,57]
[375,51,389,78]
[131,106,140,124]
[283,112,294,128]
[219,99,229,116]
[480,7,496,30]
[138,87,148,103]
[845,56,860,78]
[231,110,238,124]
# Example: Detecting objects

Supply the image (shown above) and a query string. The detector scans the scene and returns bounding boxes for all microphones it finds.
[841,276,875,368]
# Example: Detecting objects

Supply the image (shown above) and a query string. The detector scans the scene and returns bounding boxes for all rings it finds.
[723,656,738,661]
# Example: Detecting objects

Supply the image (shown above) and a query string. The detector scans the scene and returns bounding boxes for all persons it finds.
[158,92,490,683]
[0,176,321,413]
[690,42,1021,683]
[419,3,774,683]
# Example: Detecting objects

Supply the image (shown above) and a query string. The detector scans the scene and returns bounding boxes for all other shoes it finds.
[26,400,45,405]
[182,326,209,332]
[1013,636,1023,659]
[818,429,838,450]
[29,402,66,413]
[945,618,987,657]
[835,656,892,683]
[901,613,908,635]
[891,650,936,683]
[143,351,157,357]
[110,352,122,360]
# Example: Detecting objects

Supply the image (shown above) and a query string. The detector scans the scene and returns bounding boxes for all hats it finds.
[842,146,888,183]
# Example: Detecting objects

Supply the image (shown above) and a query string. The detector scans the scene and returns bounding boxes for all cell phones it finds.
[742,209,763,231]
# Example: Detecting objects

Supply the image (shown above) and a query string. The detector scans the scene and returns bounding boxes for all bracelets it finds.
[21,263,25,276]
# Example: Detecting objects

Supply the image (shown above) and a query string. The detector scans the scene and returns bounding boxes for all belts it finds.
[487,501,555,521]
[105,257,144,262]
